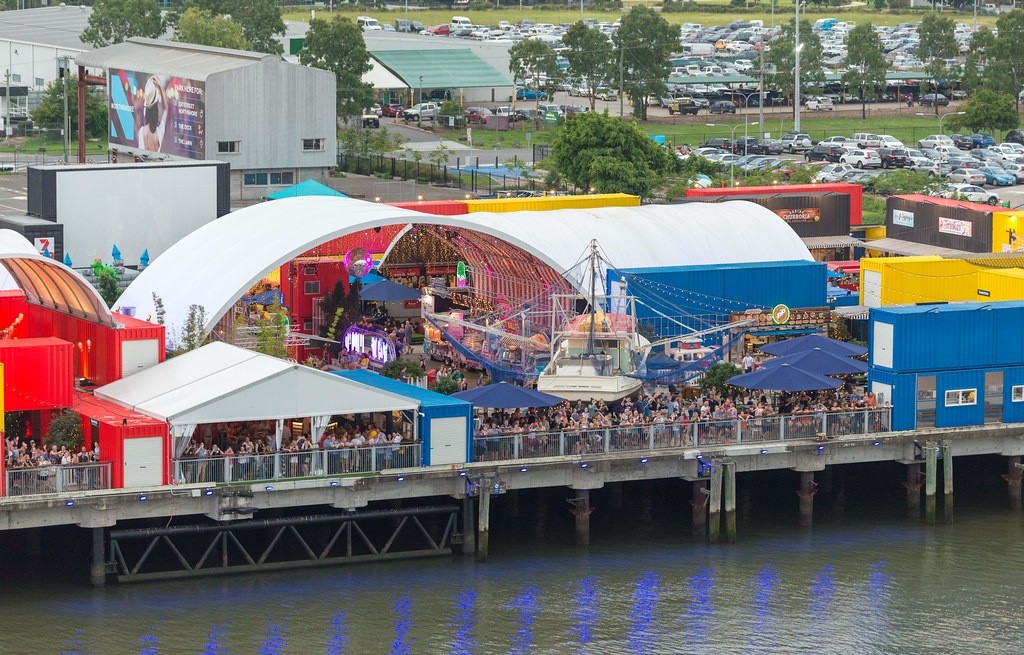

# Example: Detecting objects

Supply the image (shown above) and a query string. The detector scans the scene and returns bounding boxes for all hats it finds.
[370,431,377,437]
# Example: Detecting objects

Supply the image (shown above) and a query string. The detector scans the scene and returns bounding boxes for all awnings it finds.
[838,305,869,335]
[864,238,964,256]
[800,236,865,248]
[750,328,820,336]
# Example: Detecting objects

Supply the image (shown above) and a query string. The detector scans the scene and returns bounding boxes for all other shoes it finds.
[356,470,358,472]
[341,472,348,473]
[239,479,244,481]
[350,469,352,473]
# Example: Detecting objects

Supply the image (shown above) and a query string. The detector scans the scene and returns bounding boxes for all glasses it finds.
[30,442,35,444]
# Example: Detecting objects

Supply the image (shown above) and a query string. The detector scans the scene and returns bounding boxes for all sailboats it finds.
[537,239,643,407]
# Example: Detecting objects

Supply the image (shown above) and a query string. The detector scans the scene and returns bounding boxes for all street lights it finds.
[706,121,758,188]
[57,94,68,164]
[521,311,529,372]
[914,112,966,197]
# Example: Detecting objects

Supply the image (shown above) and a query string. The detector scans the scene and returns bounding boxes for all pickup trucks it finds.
[402,102,443,121]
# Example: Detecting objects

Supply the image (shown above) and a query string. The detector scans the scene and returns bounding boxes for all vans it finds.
[537,104,564,119]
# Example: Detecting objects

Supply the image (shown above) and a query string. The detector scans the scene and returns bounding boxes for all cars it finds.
[461,106,496,124]
[371,103,383,118]
[358,15,1004,115]
[471,189,570,200]
[673,132,1024,207]
[517,88,548,101]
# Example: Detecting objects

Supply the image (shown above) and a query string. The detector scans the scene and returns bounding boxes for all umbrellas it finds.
[450,381,566,411]
[265,180,347,199]
[726,364,843,391]
[759,334,868,357]
[760,349,867,374]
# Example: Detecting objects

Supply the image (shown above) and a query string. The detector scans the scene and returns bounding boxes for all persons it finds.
[400,355,484,389]
[4,435,99,493]
[184,417,414,482]
[742,352,753,374]
[753,356,761,369]
[307,318,414,371]
[476,383,890,460]
[840,276,851,296]
[139,76,168,152]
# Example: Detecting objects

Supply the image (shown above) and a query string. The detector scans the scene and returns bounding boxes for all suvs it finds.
[362,107,379,129]
[382,104,403,117]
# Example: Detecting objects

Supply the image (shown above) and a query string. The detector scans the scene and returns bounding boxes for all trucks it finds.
[490,107,517,122]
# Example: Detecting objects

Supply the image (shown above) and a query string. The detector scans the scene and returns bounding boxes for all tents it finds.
[646,353,681,376]
[93,341,420,483]
